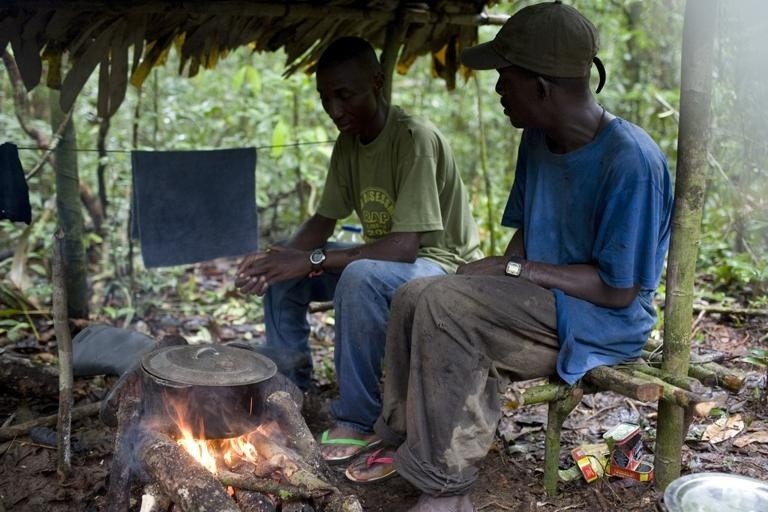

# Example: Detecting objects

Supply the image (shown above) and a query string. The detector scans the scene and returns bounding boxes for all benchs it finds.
[516,338,745,495]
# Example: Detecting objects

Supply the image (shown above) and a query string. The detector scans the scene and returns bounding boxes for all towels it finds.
[124,144,260,271]
[0,142,36,224]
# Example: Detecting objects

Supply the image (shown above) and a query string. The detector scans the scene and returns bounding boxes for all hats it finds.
[461,0,611,94]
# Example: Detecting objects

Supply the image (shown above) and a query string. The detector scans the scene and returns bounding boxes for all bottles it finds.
[335,225,367,244]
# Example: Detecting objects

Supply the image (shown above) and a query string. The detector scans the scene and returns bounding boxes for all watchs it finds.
[505,257,523,278]
[307,244,329,270]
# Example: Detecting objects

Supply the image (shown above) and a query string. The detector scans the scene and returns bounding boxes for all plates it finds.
[663,471,768,511]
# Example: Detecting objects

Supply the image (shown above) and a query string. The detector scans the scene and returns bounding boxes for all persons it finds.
[236,36,490,463]
[345,3,677,512]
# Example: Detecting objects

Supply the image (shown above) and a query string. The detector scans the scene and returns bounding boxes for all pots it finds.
[139,338,279,440]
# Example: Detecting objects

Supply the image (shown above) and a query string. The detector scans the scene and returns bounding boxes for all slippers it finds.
[345,445,407,486]
[316,426,384,466]
